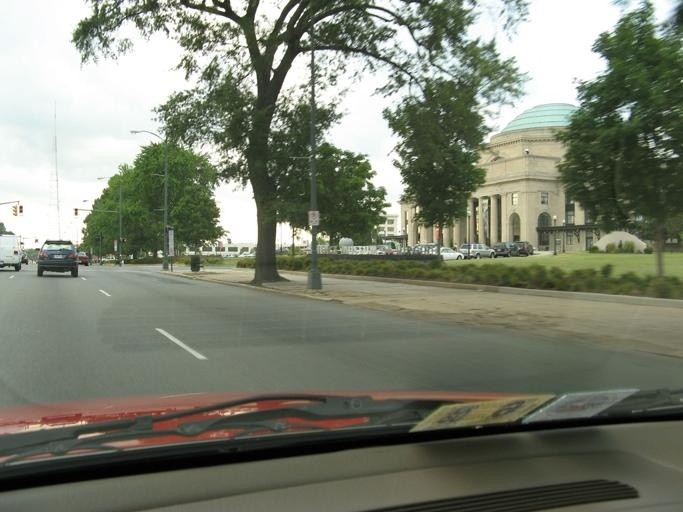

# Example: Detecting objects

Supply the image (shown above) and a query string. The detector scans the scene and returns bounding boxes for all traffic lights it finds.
[12,204,18,216]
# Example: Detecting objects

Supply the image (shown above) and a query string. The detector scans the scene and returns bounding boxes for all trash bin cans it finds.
[190,255,200,272]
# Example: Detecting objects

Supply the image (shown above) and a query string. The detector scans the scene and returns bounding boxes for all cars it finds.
[37,239,79,278]
[429,240,534,260]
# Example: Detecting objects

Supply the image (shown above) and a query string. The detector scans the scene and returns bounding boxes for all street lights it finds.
[96,176,122,255]
[130,129,169,270]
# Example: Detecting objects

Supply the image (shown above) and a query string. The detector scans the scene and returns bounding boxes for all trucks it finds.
[0,234,22,271]
